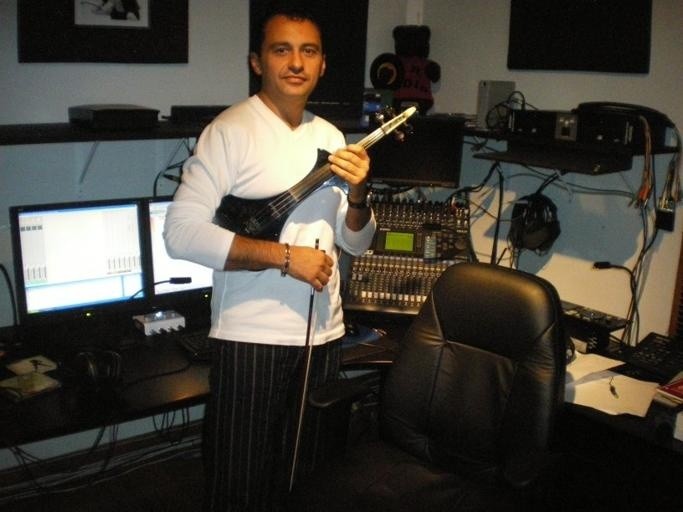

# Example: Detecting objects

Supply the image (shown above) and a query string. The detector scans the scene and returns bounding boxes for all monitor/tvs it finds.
[8,196,150,358]
[146,195,214,329]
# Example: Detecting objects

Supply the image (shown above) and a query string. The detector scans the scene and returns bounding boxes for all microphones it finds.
[595,260,638,347]
[127,276,192,309]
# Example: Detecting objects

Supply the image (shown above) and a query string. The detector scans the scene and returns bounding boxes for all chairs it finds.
[281,261,567,512]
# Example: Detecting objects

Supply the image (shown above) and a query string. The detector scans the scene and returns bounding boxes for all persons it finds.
[161,4,378,512]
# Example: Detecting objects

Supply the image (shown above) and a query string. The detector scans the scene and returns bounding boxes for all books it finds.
[656,377,683,408]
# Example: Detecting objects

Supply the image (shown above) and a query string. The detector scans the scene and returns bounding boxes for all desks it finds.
[1,299,683,455]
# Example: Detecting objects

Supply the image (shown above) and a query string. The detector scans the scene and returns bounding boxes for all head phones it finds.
[76,348,124,391]
[508,192,561,253]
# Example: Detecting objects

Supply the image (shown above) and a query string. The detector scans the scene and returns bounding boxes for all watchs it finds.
[347,186,375,209]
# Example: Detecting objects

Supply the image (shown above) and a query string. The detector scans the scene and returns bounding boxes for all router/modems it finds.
[129,308,188,336]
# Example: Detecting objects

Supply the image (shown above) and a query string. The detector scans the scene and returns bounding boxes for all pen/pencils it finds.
[610,385,619,399]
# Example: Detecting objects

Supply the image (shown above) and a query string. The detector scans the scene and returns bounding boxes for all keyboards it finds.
[176,333,214,362]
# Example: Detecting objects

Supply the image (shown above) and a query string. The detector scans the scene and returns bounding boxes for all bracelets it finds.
[280,242,291,277]
